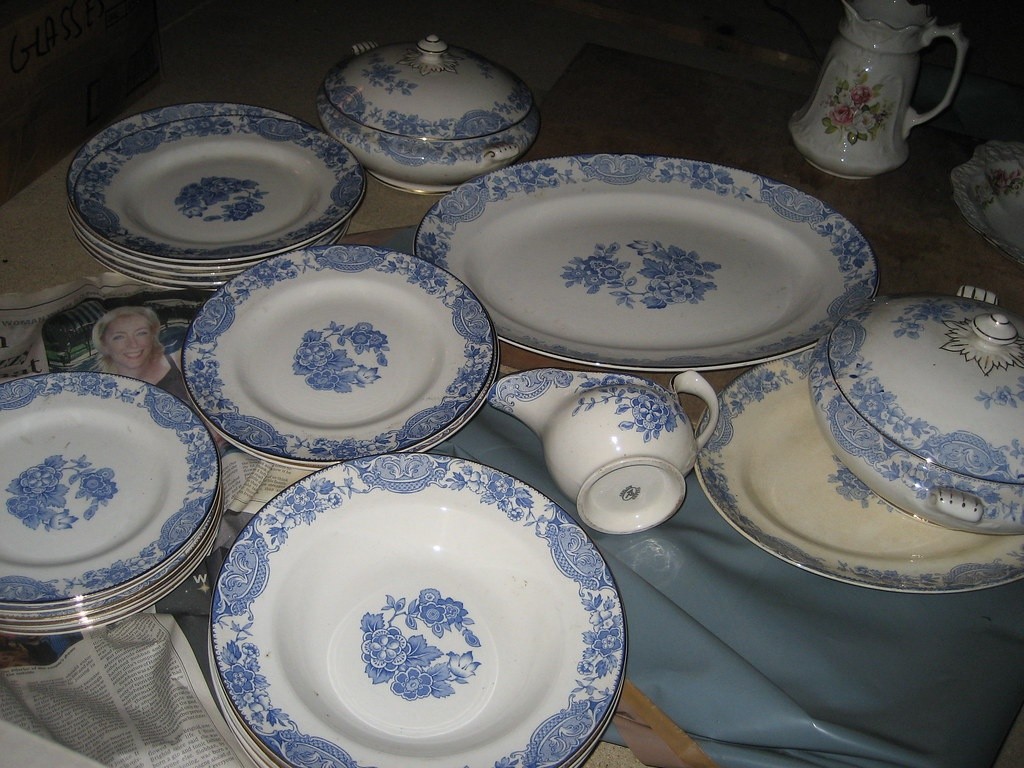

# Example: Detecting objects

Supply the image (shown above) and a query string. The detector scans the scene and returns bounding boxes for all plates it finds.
[0,3,1024,766]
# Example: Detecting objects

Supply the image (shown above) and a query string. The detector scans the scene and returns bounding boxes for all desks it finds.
[0,0,1024,768]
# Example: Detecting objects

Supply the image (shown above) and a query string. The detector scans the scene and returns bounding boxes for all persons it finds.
[88,304,227,470]
